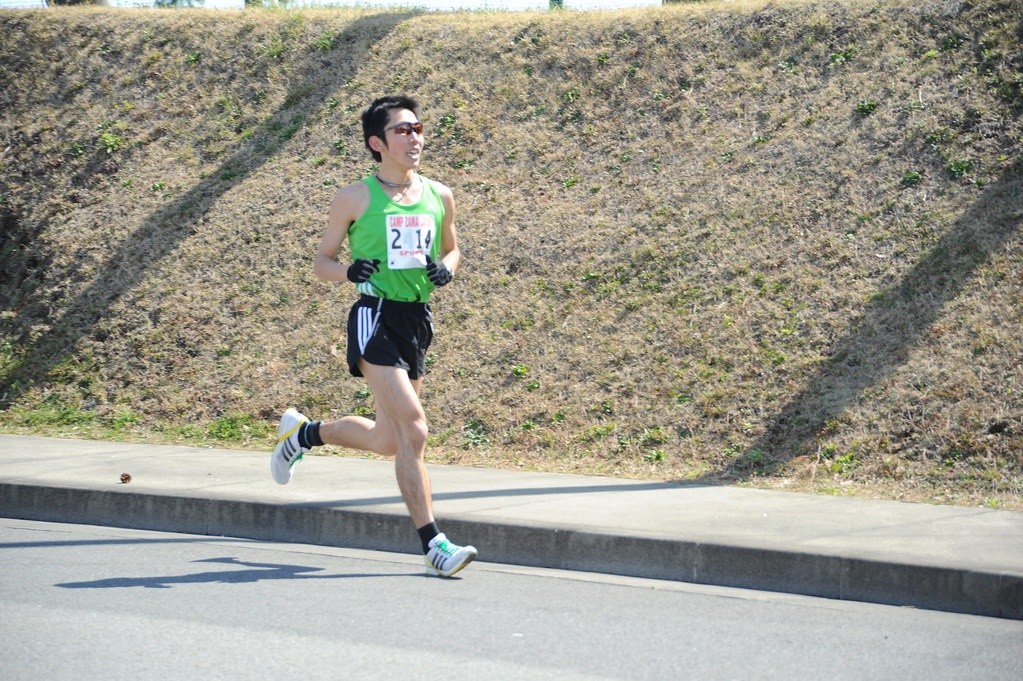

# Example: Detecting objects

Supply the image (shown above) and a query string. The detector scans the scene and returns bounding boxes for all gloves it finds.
[425,255,452,286]
[346,258,381,283]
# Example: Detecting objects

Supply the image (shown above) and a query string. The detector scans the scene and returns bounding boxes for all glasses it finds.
[376,121,422,136]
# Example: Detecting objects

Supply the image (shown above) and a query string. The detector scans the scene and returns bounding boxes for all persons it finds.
[270,96,478,577]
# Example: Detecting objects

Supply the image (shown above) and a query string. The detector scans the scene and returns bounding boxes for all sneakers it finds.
[271,408,313,485]
[425,533,479,576]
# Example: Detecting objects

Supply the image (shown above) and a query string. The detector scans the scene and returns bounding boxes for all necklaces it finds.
[376,169,417,188]
